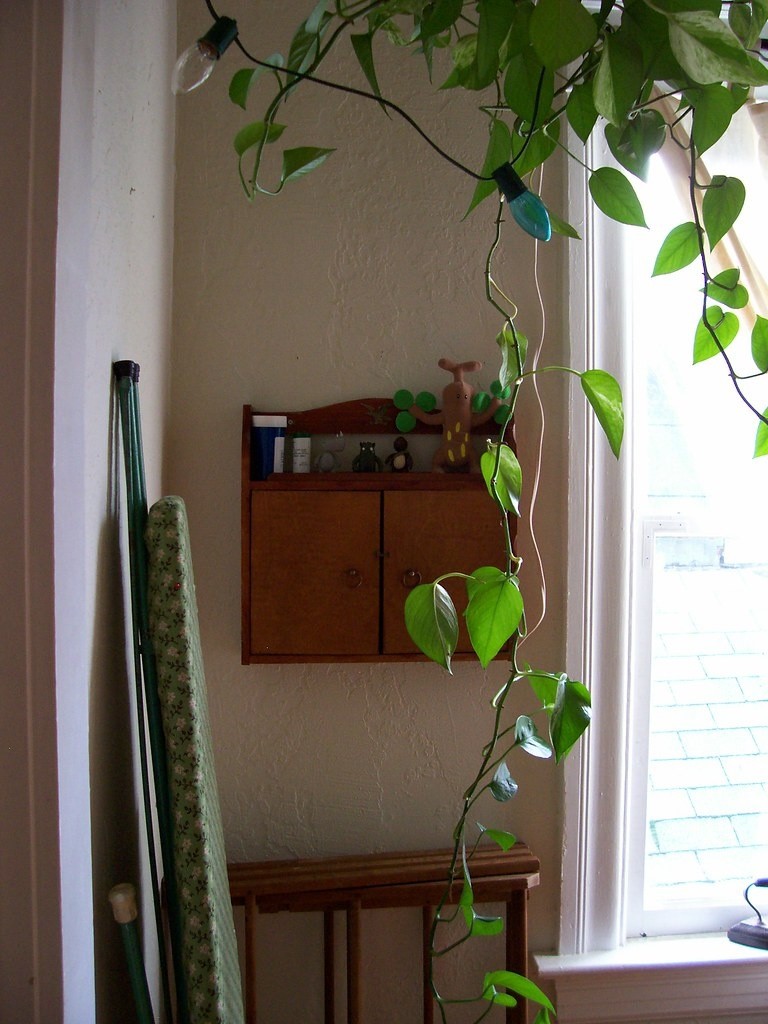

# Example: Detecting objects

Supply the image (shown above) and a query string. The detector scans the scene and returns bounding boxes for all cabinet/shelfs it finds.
[239,397,517,666]
